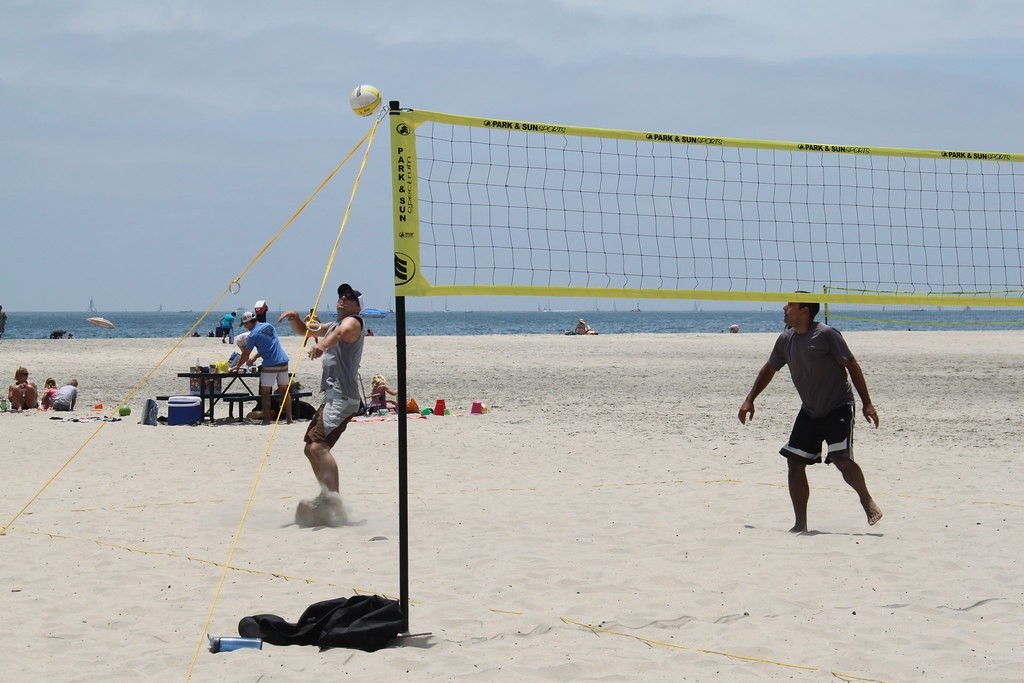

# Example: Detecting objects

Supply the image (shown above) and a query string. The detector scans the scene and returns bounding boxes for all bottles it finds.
[196,358,200,373]
[202,361,216,374]
[94,398,119,410]
[378,409,389,415]
[119,403,131,416]
[207,634,262,654]
[2,400,6,411]
[0,397,2,411]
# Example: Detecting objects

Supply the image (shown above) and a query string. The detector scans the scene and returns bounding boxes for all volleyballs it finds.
[349,84,383,117]
[119,405,131,416]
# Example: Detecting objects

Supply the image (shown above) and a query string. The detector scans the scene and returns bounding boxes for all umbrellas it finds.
[85,317,115,337]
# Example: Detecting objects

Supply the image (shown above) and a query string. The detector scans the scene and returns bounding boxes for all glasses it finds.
[339,293,357,301]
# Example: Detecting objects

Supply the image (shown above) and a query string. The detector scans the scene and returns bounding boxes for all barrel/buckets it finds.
[472,402,481,414]
[437,400,446,409]
[433,404,444,415]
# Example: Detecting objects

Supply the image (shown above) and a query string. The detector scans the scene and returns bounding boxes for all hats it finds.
[337,284,364,315]
[239,311,256,327]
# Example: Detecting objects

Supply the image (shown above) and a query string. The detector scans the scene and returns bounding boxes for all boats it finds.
[330,308,388,318]
[630,303,641,312]
[388,310,394,313]
[542,305,577,313]
[693,303,705,312]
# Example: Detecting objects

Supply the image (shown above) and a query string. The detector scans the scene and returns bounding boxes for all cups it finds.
[242,367,247,374]
[238,368,244,374]
[217,362,228,374]
[251,366,256,374]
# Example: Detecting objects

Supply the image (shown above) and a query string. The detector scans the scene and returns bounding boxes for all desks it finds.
[177,372,295,425]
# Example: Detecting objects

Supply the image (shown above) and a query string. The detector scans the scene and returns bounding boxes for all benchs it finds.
[223,391,312,422]
[156,394,250,422]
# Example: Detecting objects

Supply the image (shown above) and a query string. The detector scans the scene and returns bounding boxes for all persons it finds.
[40,378,59,409]
[303,309,321,347]
[8,367,39,412]
[220,311,236,343]
[729,324,739,333]
[738,291,882,531]
[235,330,252,362]
[575,319,589,334]
[369,375,397,414]
[50,330,66,339]
[0,304,8,338]
[230,312,293,425]
[278,283,365,492]
[52,379,78,411]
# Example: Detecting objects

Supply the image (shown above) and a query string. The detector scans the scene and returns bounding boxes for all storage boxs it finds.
[166,395,202,426]
